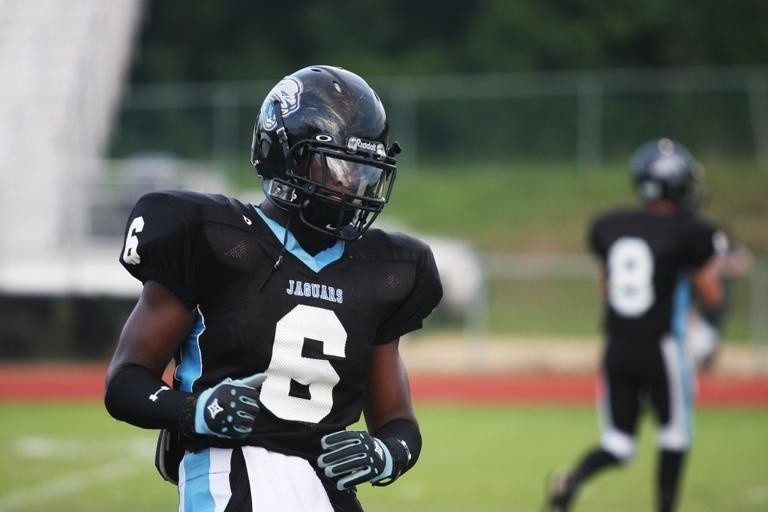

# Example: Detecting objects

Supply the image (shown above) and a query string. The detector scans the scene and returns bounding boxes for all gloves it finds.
[318,430,394,492]
[191,371,268,441]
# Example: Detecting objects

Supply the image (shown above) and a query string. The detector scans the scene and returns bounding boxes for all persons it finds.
[540,137,756,511]
[103,68,441,512]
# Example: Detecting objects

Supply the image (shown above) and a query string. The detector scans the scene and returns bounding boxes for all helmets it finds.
[251,65,405,243]
[630,136,710,213]
